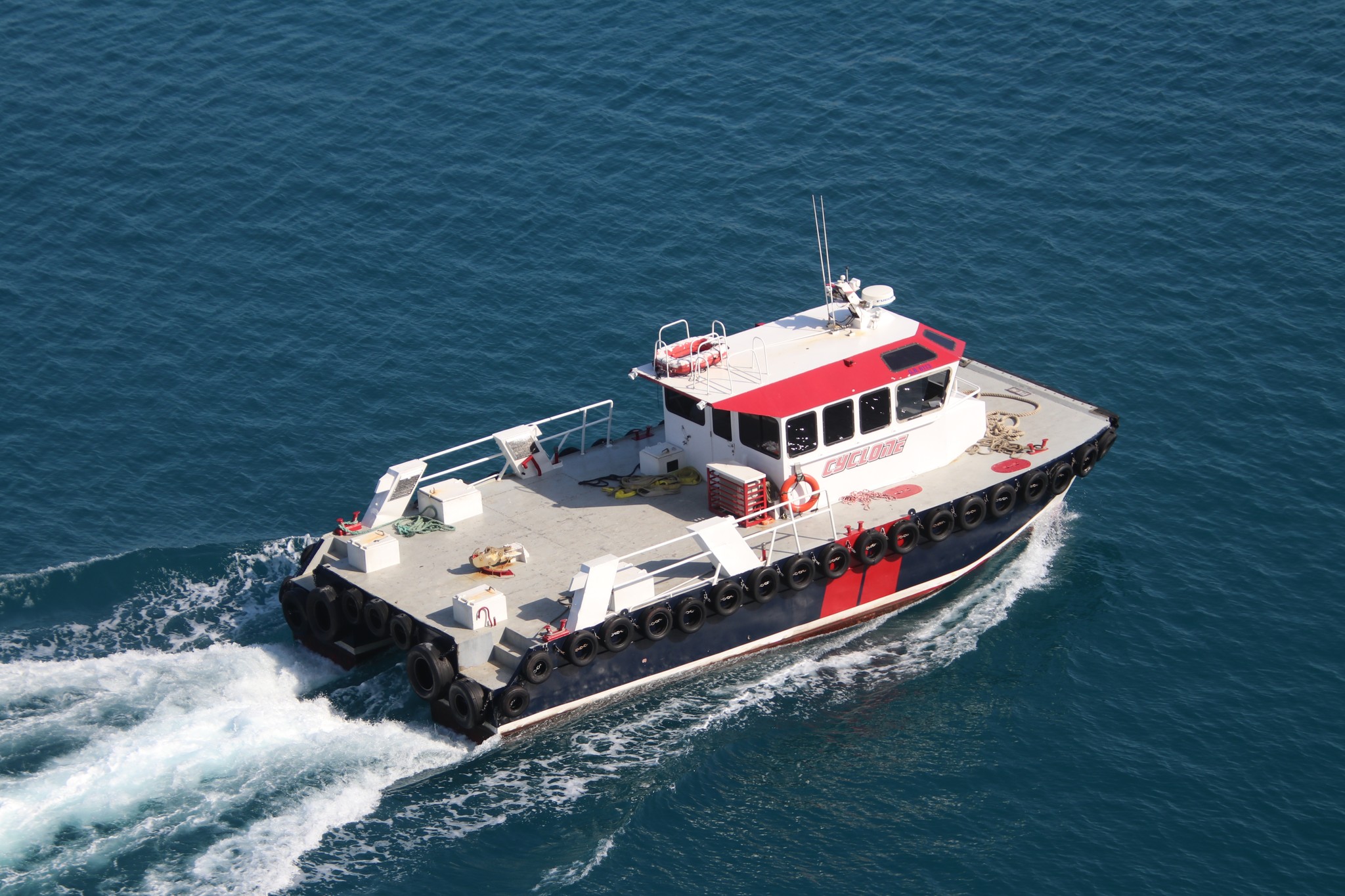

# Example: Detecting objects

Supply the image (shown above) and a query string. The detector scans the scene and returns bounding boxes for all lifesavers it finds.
[783,555,816,591]
[625,428,643,437]
[1020,470,1048,505]
[558,446,581,458]
[305,581,489,733]
[1073,443,1098,477]
[657,420,664,426]
[299,541,316,567]
[640,604,673,641]
[1048,461,1073,495]
[988,483,1016,517]
[819,543,851,579]
[523,650,553,684]
[855,530,888,565]
[601,615,634,653]
[653,336,724,376]
[591,438,612,447]
[710,580,744,617]
[674,597,707,634]
[890,520,919,555]
[1095,428,1118,462]
[274,574,291,603]
[780,474,820,512]
[923,508,954,542]
[501,687,530,718]
[956,495,986,530]
[748,567,780,603]
[564,629,599,668]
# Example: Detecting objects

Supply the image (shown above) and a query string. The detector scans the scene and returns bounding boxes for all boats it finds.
[277,193,1123,748]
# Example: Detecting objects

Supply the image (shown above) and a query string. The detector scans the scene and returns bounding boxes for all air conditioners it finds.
[719,473,761,517]
